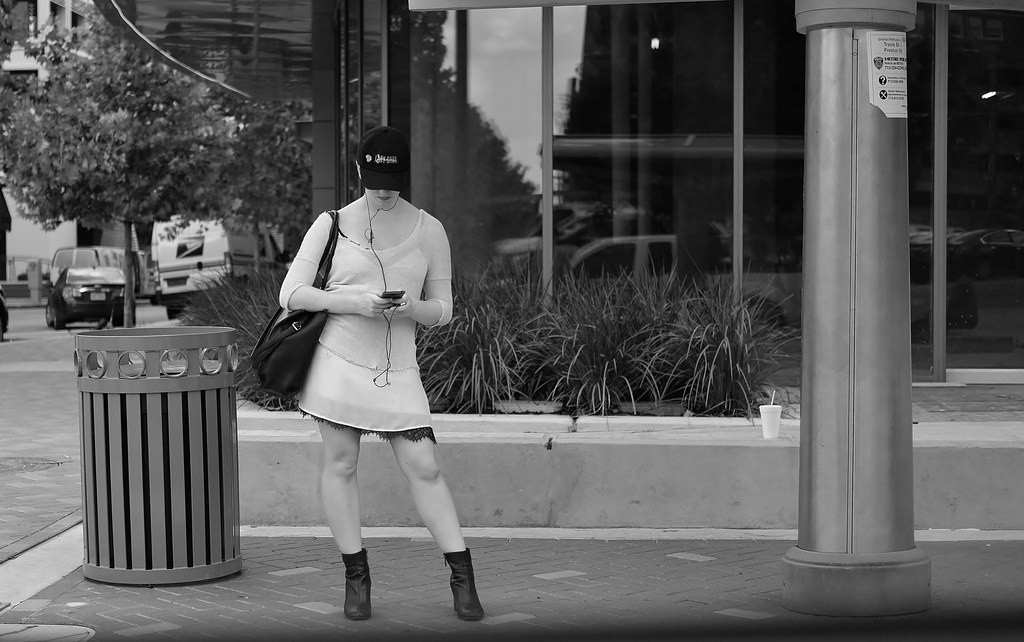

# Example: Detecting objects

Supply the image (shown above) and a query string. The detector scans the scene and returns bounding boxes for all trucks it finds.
[150,209,283,320]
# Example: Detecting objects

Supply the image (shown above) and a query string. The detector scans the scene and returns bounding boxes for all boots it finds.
[444,548,485,620]
[343,548,371,619]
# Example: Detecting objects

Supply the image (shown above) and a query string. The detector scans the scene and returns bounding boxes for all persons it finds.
[278,126,486,622]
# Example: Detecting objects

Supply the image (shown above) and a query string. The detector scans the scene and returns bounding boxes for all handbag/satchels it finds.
[250,210,339,397]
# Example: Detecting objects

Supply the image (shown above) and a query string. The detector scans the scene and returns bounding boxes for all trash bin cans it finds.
[73,327,244,586]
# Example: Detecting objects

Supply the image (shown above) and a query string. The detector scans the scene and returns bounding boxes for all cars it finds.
[489,202,803,333]
[41,265,137,327]
[45,244,162,307]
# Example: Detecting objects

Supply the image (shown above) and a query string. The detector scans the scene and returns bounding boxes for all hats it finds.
[357,125,409,191]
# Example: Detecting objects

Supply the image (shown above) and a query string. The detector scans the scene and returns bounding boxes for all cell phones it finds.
[382,291,406,299]
